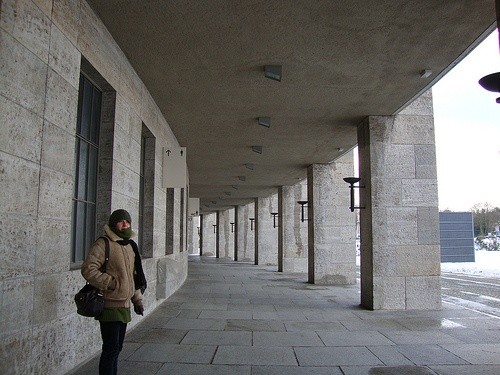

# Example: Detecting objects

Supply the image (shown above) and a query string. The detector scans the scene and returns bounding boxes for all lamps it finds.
[205,185,238,208]
[257,117,271,128]
[240,176,246,181]
[230,223,236,232]
[271,213,280,228]
[252,146,262,154]
[343,177,366,212]
[297,201,310,222]
[249,218,255,230]
[213,225,217,233]
[478,71,500,103]
[197,227,200,234]
[245,163,256,170]
[265,64,281,82]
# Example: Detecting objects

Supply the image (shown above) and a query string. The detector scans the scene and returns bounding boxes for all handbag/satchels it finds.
[75,284,104,319]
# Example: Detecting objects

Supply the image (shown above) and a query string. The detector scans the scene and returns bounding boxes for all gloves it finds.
[134,305,144,316]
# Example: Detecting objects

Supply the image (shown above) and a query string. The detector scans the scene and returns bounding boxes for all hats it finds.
[109,209,132,229]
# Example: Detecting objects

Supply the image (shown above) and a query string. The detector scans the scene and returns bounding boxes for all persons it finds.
[81,209,147,375]
